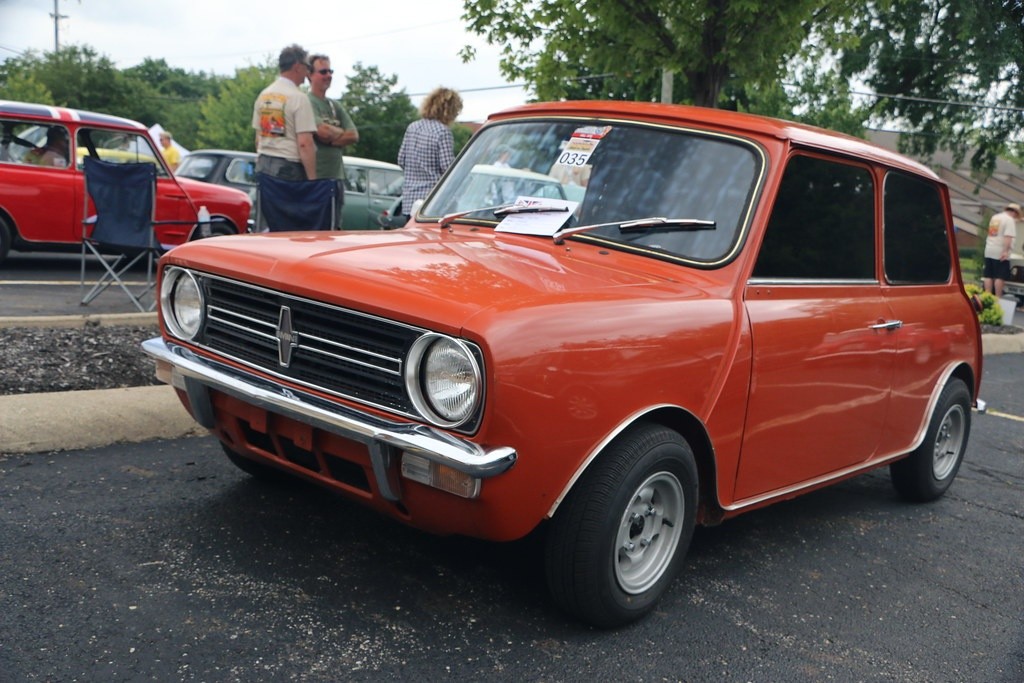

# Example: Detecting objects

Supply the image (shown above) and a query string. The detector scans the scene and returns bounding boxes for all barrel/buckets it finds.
[998,299,1017,326]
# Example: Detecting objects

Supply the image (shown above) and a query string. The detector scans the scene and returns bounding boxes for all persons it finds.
[40,127,70,167]
[252,43,318,231]
[985,202,1021,298]
[159,131,180,170]
[490,149,513,200]
[398,87,463,225]
[308,54,359,230]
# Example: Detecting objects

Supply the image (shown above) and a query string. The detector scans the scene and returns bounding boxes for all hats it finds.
[1000,203,1024,220]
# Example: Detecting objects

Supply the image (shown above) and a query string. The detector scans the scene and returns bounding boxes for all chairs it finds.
[81,155,224,314]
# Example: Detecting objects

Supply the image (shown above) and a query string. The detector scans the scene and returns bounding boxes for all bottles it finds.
[198,206,210,237]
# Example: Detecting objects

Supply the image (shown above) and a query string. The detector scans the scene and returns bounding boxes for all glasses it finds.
[302,62,312,73]
[314,69,334,74]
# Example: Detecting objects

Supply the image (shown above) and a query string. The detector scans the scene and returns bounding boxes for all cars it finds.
[137,97,987,626]
[338,153,406,232]
[9,123,158,171]
[456,161,588,232]
[0,100,252,266]
[177,151,271,234]
